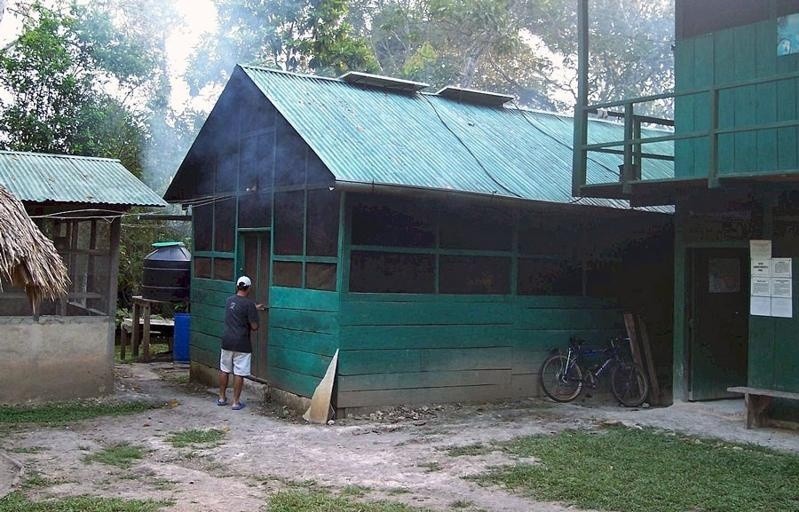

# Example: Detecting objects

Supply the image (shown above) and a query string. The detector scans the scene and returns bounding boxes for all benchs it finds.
[727,386,799,430]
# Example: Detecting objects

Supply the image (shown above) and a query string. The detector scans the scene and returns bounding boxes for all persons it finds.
[215,274,266,410]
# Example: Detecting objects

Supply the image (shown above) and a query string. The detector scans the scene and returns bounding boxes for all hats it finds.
[236,274,251,289]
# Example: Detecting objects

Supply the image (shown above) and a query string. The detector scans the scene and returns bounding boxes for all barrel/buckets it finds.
[171,311,190,362]
[141,238,191,313]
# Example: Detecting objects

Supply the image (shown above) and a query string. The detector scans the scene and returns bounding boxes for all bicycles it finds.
[536,336,651,408]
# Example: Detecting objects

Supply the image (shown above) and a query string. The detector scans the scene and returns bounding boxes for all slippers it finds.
[231,401,245,410]
[216,397,228,404]
